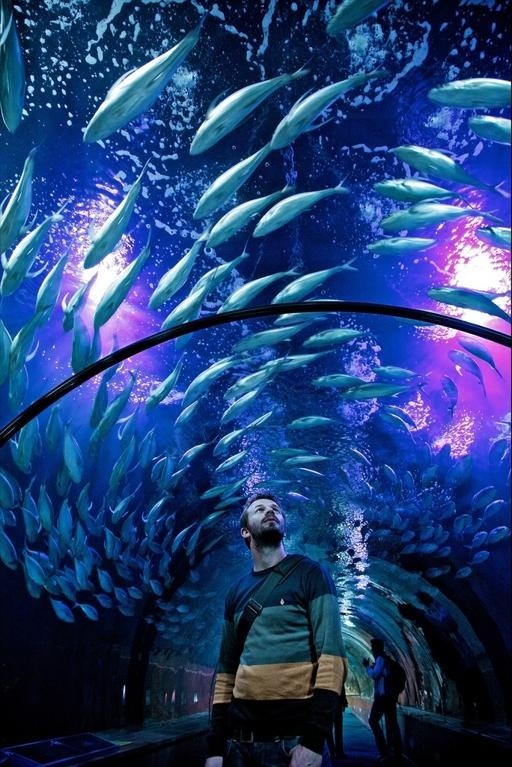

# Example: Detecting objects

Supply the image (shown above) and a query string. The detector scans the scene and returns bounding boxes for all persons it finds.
[202,488,344,765]
[359,635,405,759]
[326,657,350,759]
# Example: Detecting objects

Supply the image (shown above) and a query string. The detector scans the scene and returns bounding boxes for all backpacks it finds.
[375,654,406,692]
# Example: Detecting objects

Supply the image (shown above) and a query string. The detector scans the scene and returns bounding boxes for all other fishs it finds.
[1,0,512,671]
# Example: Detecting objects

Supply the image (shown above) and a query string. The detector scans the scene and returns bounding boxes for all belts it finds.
[224,724,296,745]
